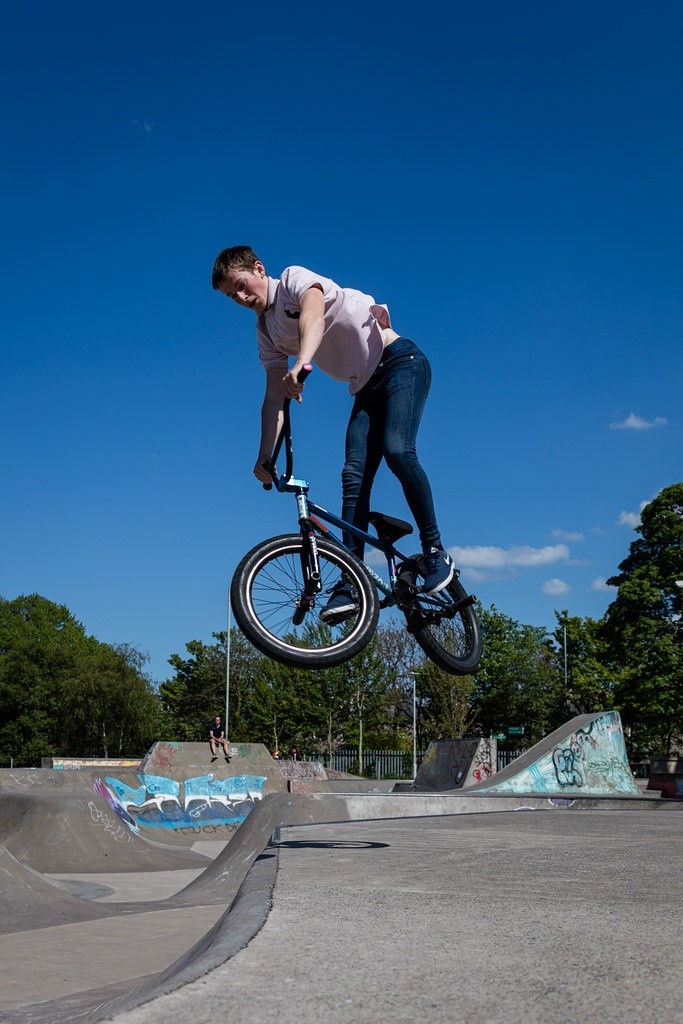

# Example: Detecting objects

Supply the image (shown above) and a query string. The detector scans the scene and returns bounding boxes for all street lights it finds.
[564,620,571,686]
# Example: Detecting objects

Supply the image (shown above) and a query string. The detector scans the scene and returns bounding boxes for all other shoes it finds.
[225,755,232,759]
[212,753,218,759]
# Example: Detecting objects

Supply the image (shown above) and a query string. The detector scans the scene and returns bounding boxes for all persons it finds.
[292,747,301,761]
[211,246,455,622]
[208,716,232,759]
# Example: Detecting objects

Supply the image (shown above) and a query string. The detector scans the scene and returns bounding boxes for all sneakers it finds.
[320,580,357,622]
[423,546,454,595]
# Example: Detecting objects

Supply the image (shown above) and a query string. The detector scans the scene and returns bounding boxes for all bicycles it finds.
[229,363,485,676]
